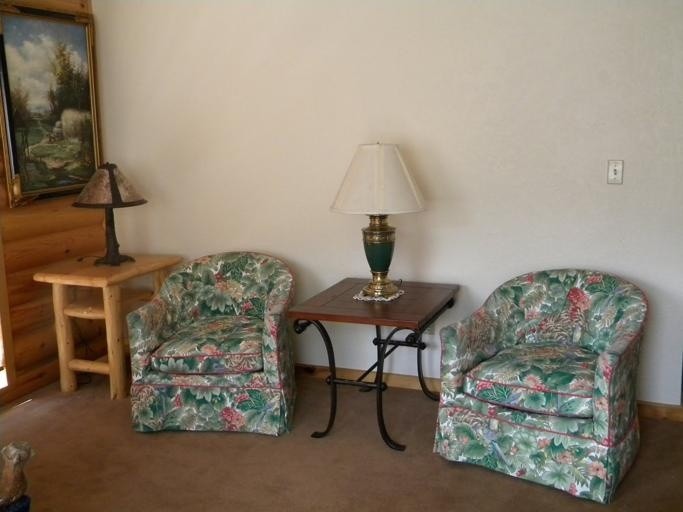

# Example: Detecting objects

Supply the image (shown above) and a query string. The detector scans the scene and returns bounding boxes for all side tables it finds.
[287,277,461,452]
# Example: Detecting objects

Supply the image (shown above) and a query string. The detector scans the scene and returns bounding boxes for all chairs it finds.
[125,250,295,439]
[431,267,649,505]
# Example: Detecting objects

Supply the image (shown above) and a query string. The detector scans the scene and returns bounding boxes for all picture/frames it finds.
[0,2,103,210]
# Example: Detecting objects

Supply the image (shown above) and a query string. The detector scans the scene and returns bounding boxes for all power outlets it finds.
[607,160,623,184]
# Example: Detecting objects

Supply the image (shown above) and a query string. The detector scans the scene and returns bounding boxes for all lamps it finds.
[71,162,148,267]
[329,143,425,298]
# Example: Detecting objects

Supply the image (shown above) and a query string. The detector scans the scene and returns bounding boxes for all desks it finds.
[32,251,183,401]
